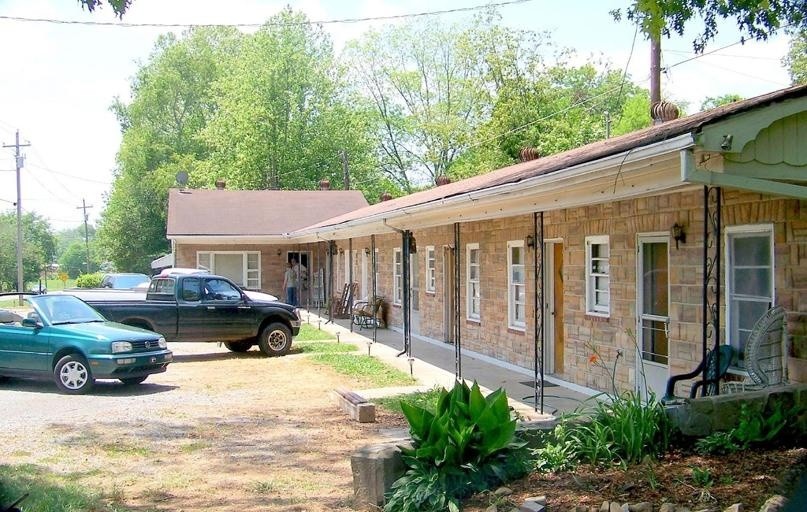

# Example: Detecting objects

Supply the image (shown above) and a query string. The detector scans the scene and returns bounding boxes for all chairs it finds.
[324,284,385,331]
[661,306,790,406]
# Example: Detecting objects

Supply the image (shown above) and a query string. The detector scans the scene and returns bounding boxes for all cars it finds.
[29,285,47,294]
[0,293,173,395]
[100,272,154,290]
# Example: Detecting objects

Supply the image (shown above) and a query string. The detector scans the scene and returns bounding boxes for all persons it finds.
[283,263,298,306]
[289,257,306,305]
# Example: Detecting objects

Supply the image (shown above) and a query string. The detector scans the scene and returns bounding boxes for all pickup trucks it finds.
[53,269,301,355]
